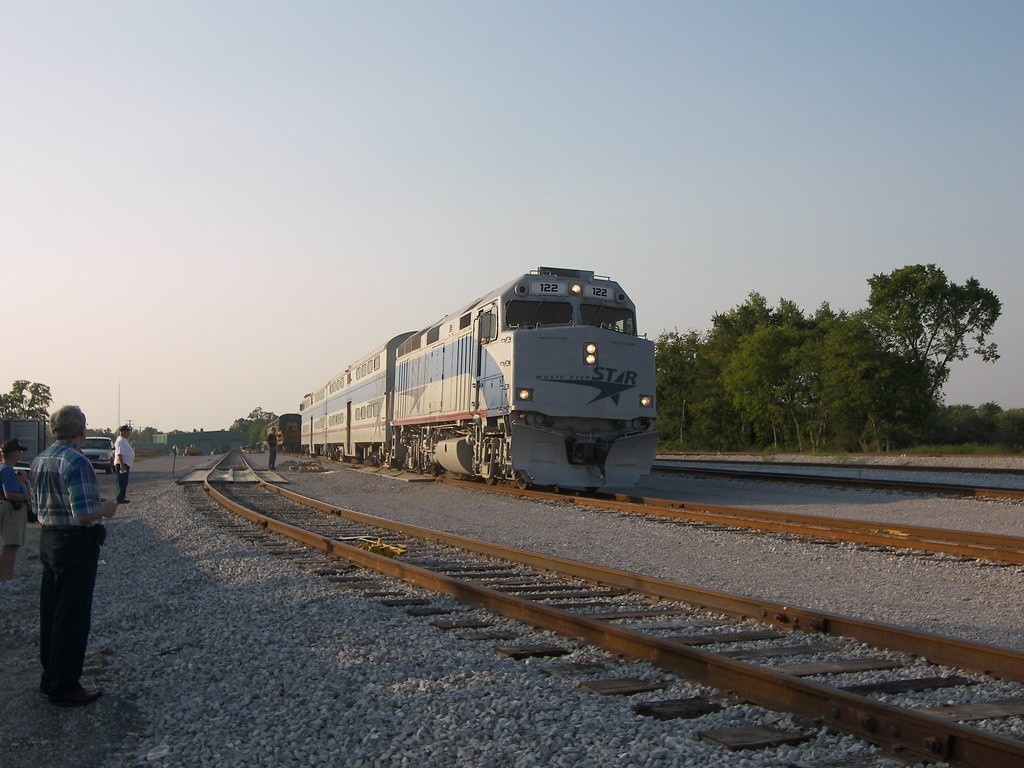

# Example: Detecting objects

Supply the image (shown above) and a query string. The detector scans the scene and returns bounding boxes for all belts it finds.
[40,525,94,532]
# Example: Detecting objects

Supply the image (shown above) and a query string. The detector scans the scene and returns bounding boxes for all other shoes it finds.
[269,466,276,470]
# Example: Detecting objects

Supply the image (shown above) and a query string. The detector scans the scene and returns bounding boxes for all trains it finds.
[264,414,301,454]
[299,266,656,499]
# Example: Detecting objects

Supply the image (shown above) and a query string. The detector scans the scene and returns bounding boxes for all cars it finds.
[81,436,116,474]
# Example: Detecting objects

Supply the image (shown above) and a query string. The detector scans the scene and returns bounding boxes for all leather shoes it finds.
[117,499,131,504]
[40,682,104,706]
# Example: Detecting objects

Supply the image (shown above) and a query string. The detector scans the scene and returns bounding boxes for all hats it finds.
[0,438,28,452]
[119,425,131,431]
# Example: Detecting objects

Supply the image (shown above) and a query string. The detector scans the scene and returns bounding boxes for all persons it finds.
[0,438,31,592]
[114,426,135,504]
[29,406,119,707]
[16,470,38,523]
[267,427,277,470]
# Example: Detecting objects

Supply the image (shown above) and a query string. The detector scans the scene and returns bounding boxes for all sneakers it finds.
[0,579,22,593]
